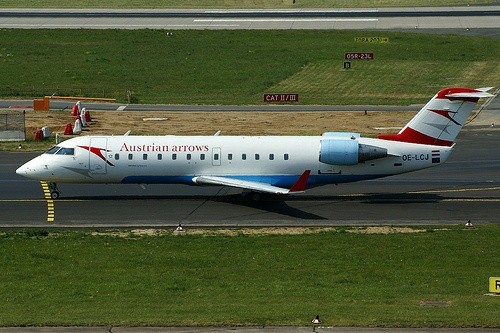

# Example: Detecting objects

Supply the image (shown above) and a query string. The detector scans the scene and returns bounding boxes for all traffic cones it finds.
[35,126,50,140]
[64,100,91,135]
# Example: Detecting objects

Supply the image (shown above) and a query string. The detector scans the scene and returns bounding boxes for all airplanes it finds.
[16,86,495,200]
